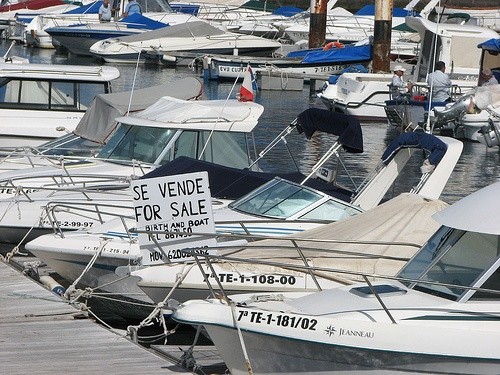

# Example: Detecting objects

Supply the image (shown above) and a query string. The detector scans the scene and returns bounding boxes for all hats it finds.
[482,66,492,75]
[394,65,406,71]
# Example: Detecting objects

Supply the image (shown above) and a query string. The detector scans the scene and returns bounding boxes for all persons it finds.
[99,0,111,23]
[427,61,455,103]
[123,0,142,17]
[392,66,406,94]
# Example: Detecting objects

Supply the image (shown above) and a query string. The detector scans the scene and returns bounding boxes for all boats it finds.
[0,0,500,375]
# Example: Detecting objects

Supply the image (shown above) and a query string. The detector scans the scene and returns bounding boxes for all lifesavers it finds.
[323,41,343,51]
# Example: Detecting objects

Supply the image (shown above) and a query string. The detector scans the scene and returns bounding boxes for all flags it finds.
[240,65,257,102]
